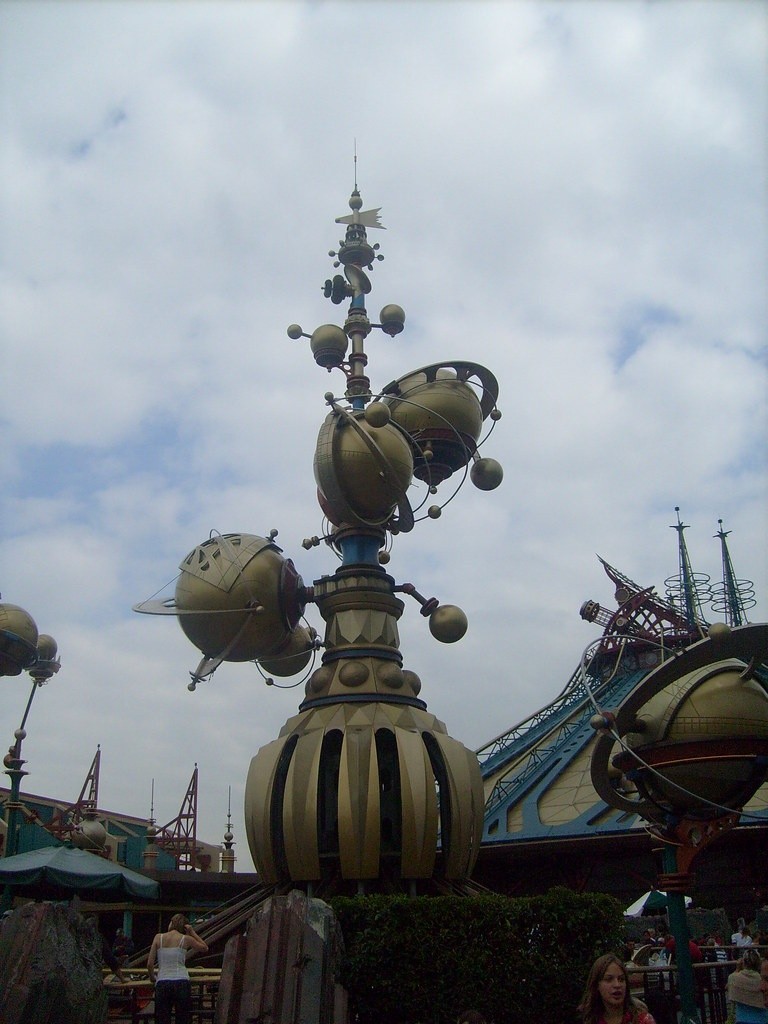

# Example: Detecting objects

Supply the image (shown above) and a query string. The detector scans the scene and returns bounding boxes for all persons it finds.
[617,903,768,1024]
[84,917,134,984]
[575,952,657,1024]
[146,913,209,1024]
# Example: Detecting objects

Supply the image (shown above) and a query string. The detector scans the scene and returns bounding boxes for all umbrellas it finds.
[623,886,692,918]
[0,841,162,910]
